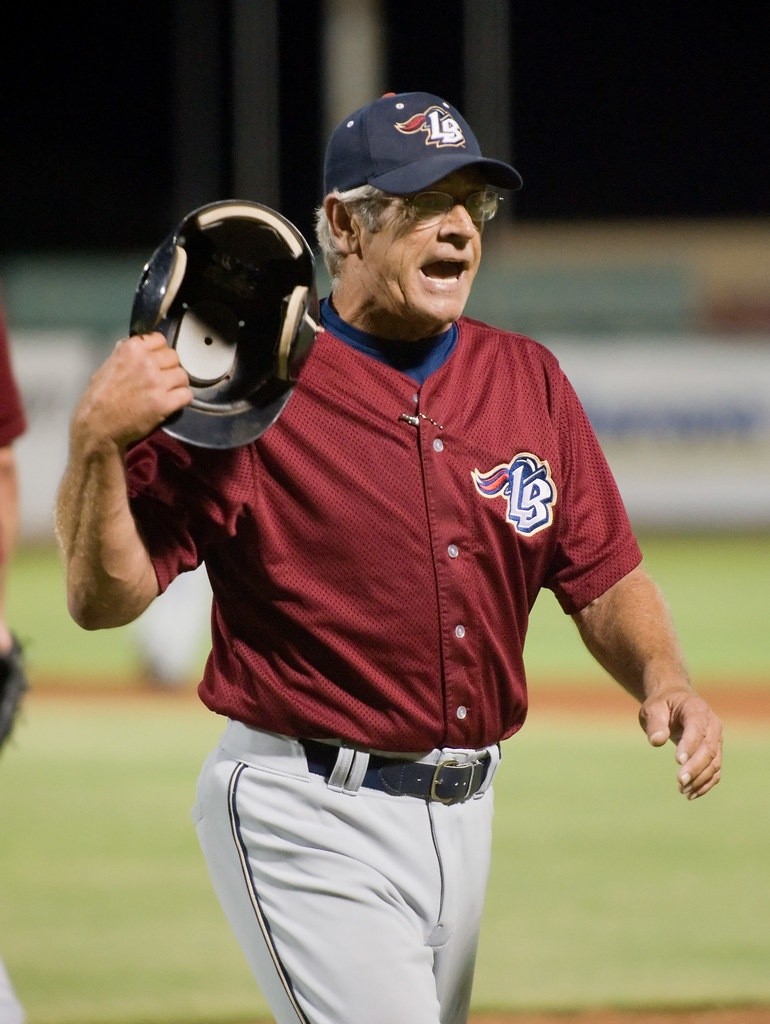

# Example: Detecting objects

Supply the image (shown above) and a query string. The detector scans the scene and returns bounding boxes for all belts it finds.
[302,737,502,806]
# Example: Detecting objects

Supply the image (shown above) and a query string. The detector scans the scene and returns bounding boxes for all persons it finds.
[0,300,30,1024]
[52,92,725,1024]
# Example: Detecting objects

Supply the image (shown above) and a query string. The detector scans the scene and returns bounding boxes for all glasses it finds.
[363,189,504,224]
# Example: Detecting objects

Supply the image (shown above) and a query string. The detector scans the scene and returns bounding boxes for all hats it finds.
[322,88,523,199]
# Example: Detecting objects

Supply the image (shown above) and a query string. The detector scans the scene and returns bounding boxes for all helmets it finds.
[125,197,321,453]
